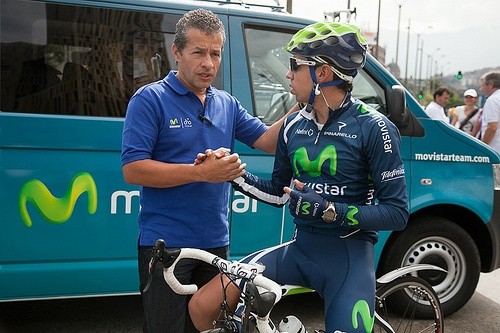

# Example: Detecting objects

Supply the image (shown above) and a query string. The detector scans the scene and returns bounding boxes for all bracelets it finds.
[299,102,305,109]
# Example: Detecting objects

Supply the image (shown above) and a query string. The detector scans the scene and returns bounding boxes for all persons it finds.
[121,9,306,333]
[470,71,500,155]
[425,87,450,124]
[188,20,410,333]
[451,88,482,138]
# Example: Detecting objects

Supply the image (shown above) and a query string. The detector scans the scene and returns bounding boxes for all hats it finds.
[463,89,477,97]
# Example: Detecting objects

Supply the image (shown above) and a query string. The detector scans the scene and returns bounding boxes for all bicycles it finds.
[144,238,448,333]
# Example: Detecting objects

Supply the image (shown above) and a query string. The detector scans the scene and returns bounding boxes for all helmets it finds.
[285,21,367,71]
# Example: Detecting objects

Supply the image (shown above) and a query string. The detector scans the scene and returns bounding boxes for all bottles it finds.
[279,315,308,333]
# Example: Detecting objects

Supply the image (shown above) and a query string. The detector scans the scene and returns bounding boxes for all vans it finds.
[0,0,500,321]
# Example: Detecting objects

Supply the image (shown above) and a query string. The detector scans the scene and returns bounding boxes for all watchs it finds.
[321,201,338,223]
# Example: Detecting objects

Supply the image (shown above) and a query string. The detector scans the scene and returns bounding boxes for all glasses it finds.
[289,57,328,71]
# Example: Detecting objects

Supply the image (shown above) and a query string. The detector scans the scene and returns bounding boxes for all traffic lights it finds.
[457,71,462,79]
[417,92,423,99]
